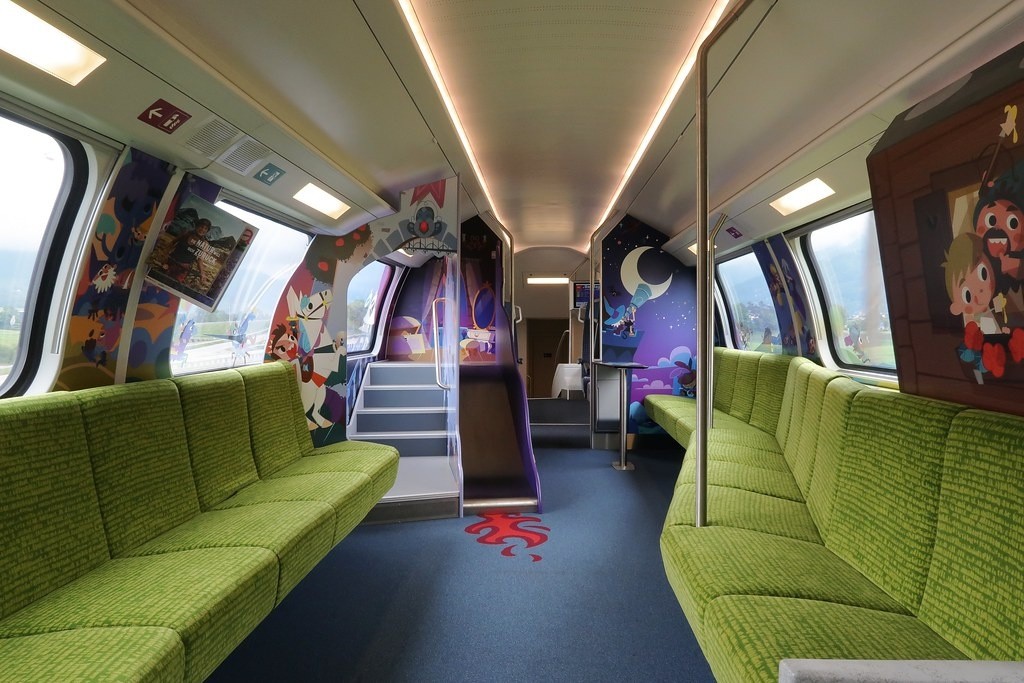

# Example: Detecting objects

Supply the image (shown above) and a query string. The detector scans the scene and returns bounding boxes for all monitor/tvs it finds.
[574,283,600,309]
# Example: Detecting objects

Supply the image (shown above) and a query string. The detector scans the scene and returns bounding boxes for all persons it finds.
[169,219,211,283]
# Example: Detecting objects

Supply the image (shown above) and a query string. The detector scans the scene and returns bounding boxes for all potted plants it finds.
[625,415,639,450]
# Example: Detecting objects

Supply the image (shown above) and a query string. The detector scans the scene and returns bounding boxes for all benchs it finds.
[644,347,1024,682]
[0,360,400,683]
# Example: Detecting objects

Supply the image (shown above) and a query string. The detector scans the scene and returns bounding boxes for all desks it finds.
[594,361,649,472]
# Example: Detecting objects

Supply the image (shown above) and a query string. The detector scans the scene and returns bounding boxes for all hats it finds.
[195,218,212,232]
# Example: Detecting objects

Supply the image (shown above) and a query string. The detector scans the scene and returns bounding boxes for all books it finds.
[144,193,259,313]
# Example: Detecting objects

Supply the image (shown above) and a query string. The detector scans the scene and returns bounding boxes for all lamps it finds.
[523,271,576,286]
